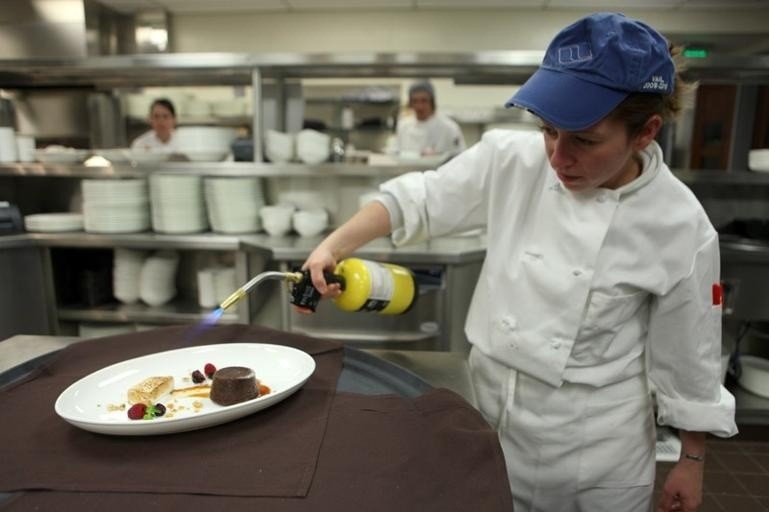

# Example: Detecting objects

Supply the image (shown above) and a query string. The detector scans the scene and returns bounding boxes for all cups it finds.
[196,268,236,311]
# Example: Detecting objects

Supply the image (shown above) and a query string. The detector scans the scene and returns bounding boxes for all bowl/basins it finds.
[259,128,330,240]
[747,148,768,171]
[111,250,179,307]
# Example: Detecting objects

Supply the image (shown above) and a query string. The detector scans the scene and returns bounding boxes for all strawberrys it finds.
[128,403,157,421]
[204,363,216,377]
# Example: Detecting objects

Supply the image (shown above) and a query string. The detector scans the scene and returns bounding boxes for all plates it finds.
[54,342,318,436]
[1,126,262,237]
[737,357,769,400]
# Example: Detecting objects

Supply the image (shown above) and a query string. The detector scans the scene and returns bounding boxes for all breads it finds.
[209,365,261,407]
[126,374,173,403]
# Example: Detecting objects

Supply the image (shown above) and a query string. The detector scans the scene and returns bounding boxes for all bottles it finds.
[341,104,356,130]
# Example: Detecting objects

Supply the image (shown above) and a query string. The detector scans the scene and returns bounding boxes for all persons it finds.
[394,80,467,168]
[294,8,741,511]
[129,98,204,162]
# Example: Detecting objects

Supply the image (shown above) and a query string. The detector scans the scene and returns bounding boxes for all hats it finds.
[504,11,677,133]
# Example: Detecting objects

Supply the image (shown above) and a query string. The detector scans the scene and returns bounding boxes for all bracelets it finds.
[679,451,705,462]
